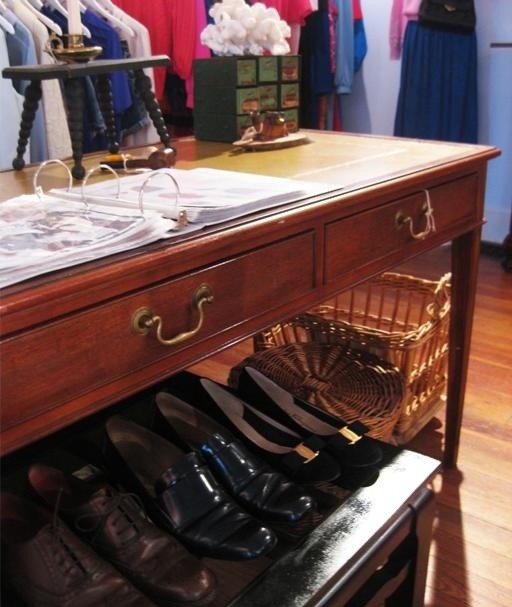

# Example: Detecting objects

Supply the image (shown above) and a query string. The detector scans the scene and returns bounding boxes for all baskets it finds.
[253,273,452,446]
[228,341,406,445]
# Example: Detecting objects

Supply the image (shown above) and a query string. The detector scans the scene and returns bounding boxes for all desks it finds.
[1,130,502,472]
[0,371,445,606]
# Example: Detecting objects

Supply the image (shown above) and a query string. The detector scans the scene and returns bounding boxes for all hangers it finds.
[0,0,135,40]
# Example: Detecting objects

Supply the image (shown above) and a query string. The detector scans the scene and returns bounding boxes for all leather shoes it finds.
[101,415,282,564]
[144,392,318,527]
[25,457,219,607]
[1,493,153,607]
[191,369,343,487]
[233,363,384,468]
